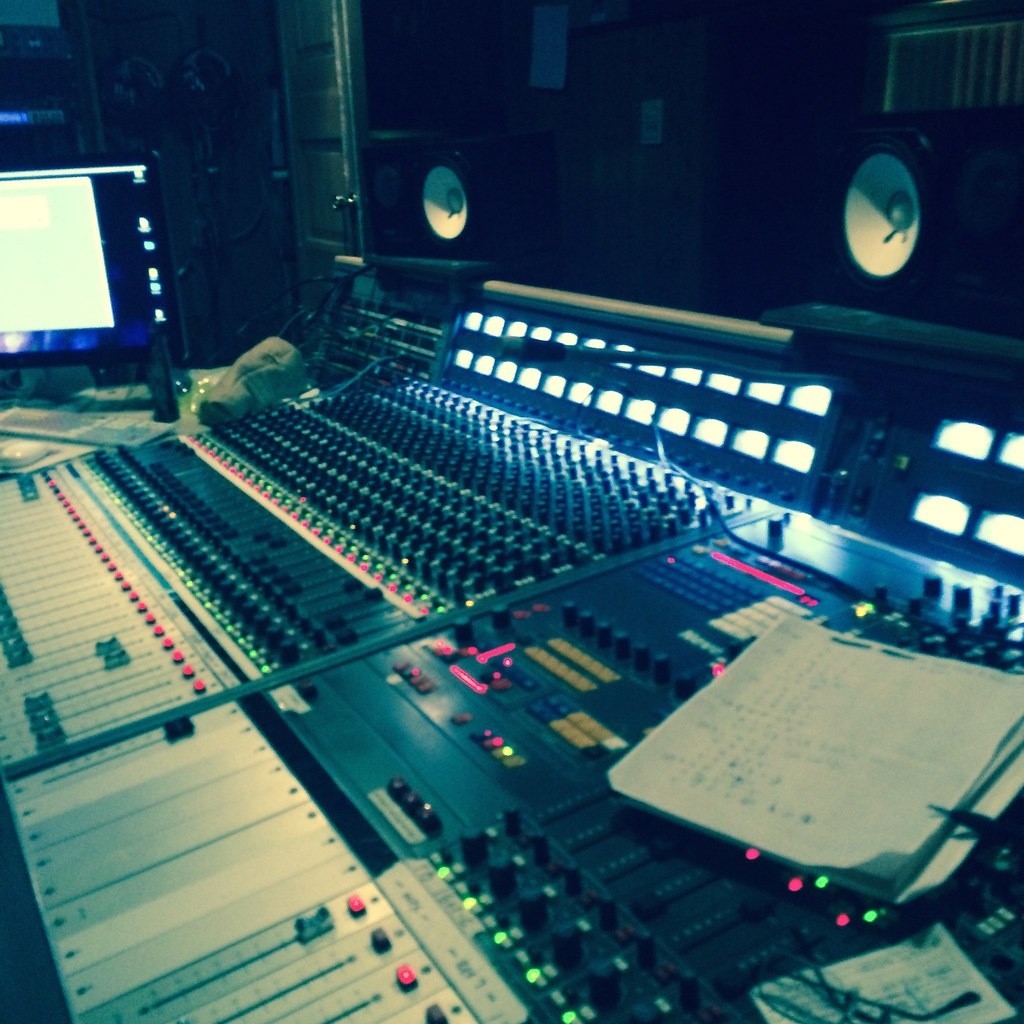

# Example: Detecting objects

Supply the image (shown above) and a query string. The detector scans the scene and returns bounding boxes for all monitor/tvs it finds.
[0,153,190,369]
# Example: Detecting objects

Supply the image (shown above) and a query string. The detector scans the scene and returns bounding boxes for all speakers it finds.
[804,105,1024,305]
[383,89,557,277]
